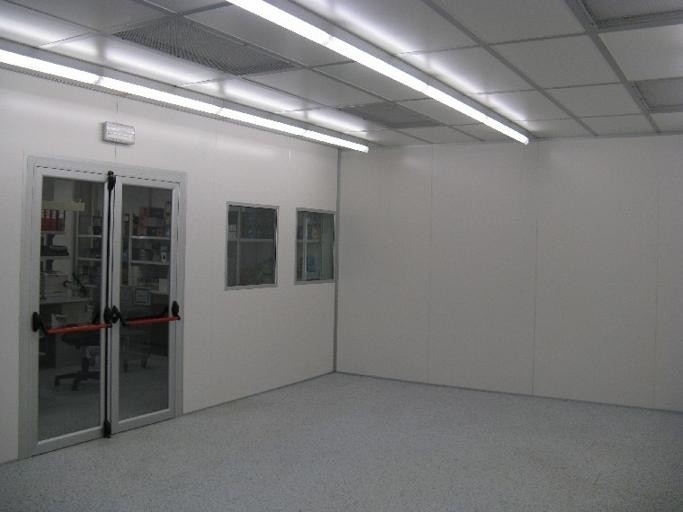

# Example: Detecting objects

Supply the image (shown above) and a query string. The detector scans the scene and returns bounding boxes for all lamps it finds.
[224,0,532,146]
[0,38,370,154]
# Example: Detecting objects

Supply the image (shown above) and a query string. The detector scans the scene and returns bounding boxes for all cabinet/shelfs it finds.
[75,209,170,294]
[40,199,92,304]
[227,207,334,286]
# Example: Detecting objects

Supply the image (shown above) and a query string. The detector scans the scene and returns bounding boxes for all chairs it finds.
[54,304,167,391]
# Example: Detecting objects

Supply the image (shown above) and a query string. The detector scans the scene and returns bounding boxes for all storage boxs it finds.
[41,271,70,299]
[137,207,164,235]
